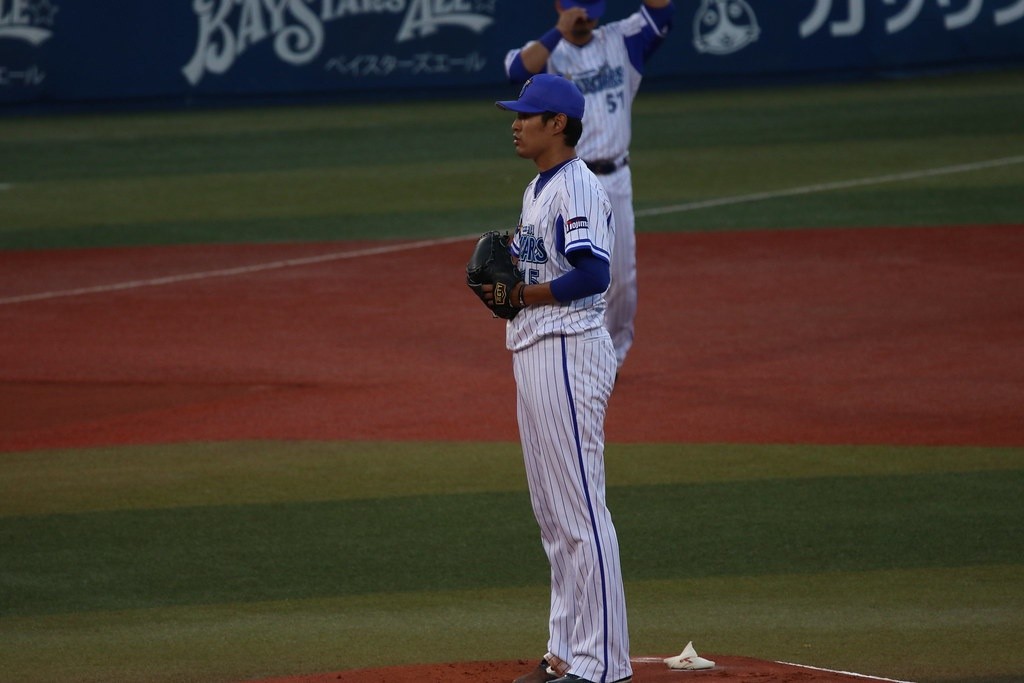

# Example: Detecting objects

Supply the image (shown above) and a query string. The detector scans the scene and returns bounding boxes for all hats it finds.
[496,75,584,119]
[561,0,606,20]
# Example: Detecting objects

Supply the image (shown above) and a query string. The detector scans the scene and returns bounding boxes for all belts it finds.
[585,157,629,174]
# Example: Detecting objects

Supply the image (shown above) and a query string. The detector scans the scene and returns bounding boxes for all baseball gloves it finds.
[467,232,523,323]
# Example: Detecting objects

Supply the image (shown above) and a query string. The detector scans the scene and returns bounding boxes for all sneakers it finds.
[512,660,561,683]
[546,671,633,683]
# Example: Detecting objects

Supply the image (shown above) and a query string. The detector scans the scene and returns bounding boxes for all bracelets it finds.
[518,284,528,307]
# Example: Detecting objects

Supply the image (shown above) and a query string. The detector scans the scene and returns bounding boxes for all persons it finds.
[504,0,677,383]
[466,74,634,683]
